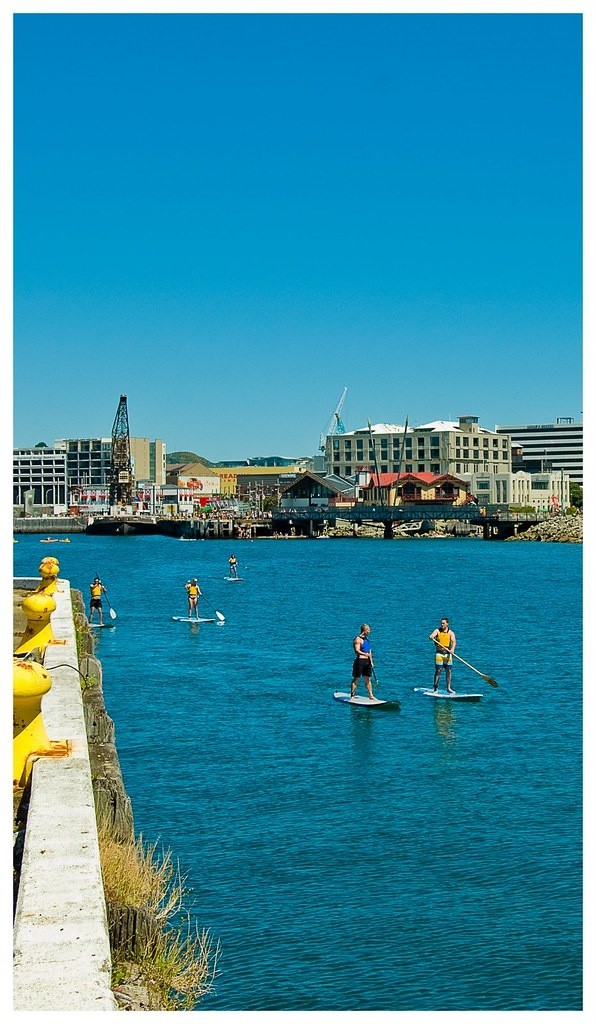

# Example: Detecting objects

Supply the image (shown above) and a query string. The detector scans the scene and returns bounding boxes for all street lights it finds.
[46,488,53,515]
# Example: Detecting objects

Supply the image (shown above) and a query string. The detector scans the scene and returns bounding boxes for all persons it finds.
[349,624,379,703]
[428,617,457,695]
[89,577,107,625]
[228,553,239,579]
[184,578,202,621]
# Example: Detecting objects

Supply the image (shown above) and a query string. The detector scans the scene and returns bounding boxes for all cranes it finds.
[317,387,348,452]
[106,394,134,515]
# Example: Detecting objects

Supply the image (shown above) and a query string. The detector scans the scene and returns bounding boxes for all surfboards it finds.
[333,689,402,708]
[88,622,115,628]
[172,615,216,623]
[413,686,483,701]
[223,576,244,582]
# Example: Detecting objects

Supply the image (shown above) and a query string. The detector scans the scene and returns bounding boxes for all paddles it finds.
[237,564,250,570]
[189,581,226,621]
[95,570,118,620]
[432,638,499,688]
[368,656,381,685]
